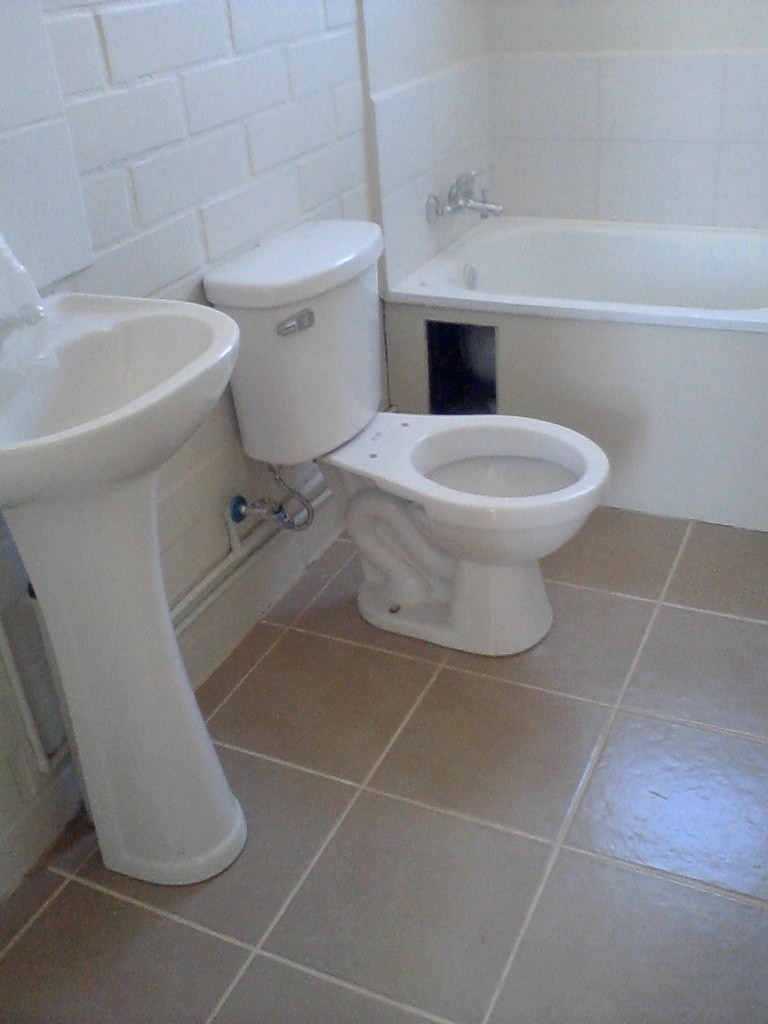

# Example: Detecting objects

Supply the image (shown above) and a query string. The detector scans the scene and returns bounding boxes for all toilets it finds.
[201,215,612,657]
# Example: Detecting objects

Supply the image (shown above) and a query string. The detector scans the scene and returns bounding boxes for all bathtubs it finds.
[388,213,768,533]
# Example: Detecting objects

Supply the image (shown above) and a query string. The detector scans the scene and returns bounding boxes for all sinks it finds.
[0,222,243,515]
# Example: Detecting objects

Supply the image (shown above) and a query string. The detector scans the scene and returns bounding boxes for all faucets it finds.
[438,188,504,219]
